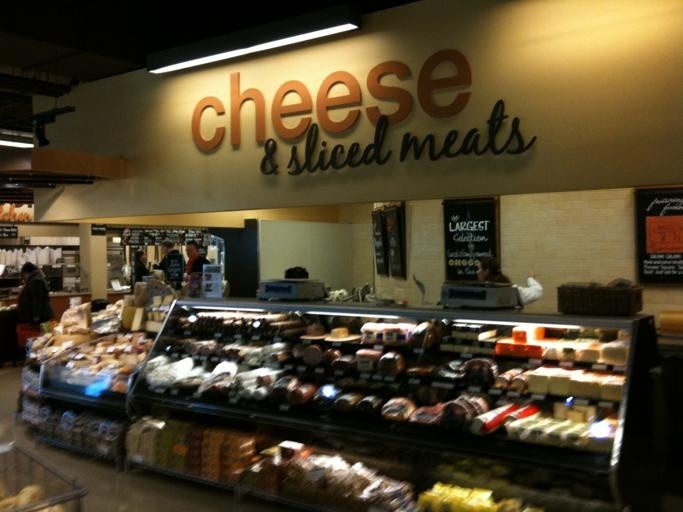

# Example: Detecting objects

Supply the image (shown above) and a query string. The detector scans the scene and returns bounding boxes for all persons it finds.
[152,240,187,290]
[17,262,53,326]
[476,263,544,305]
[134,249,151,282]
[186,240,210,274]
[285,267,308,279]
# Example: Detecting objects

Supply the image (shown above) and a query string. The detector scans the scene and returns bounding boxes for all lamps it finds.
[35,124,50,148]
[0,129,36,149]
[146,19,363,75]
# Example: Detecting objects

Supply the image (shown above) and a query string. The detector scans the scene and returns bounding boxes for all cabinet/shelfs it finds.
[16,292,666,511]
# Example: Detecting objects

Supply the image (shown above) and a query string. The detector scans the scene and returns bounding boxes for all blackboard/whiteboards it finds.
[123,228,210,249]
[2,225,17,240]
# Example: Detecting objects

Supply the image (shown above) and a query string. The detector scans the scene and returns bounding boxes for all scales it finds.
[438,278,519,312]
[258,279,328,301]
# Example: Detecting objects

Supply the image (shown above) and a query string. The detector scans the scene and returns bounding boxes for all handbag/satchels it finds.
[16,324,42,346]
